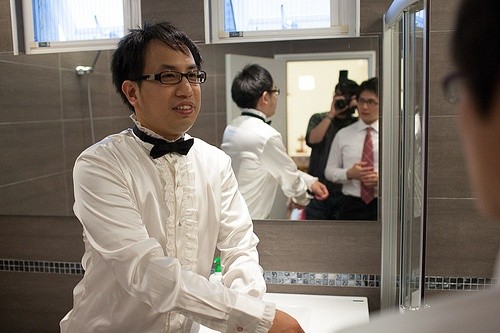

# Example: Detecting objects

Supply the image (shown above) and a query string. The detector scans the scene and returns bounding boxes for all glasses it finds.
[140,69,207,86]
[444,70,460,106]
[270,88,280,95]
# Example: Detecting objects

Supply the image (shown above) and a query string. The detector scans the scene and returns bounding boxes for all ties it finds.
[361,128,375,204]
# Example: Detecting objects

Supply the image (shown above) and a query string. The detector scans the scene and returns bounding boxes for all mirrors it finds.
[0,0,384,224]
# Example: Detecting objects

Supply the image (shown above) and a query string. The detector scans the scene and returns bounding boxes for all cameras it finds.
[336,86,352,108]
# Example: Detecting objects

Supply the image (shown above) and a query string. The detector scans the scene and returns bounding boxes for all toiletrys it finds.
[209,257,223,281]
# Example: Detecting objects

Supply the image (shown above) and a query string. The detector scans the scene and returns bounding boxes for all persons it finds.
[59,20,304,333]
[305,80,359,220]
[328,0,500,333]
[325,78,378,221]
[221,65,329,219]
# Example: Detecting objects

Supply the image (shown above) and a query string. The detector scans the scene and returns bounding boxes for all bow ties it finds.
[132,124,195,158]
[240,112,271,125]
